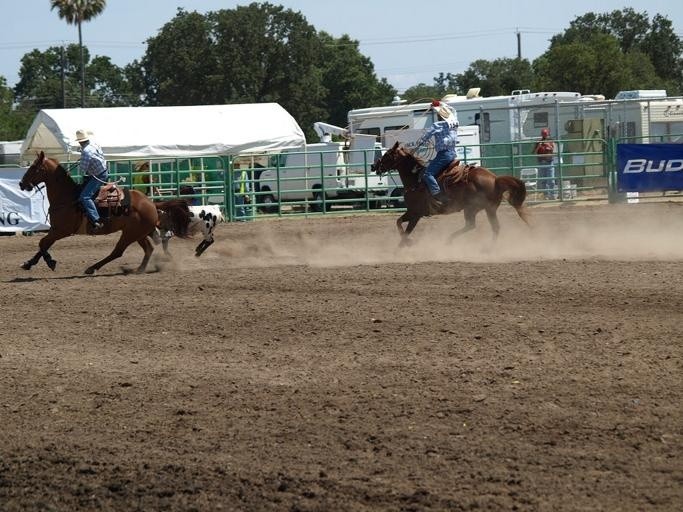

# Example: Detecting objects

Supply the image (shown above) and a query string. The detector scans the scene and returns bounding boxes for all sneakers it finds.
[434,193,447,203]
[93,218,103,229]
[545,195,557,200]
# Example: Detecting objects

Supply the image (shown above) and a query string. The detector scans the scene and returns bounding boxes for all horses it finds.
[18,150,194,275]
[370,140,531,256]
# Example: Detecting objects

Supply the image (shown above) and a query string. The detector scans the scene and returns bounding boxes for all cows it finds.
[152,204,226,257]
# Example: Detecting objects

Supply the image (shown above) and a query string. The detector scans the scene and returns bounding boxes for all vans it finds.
[258,141,389,214]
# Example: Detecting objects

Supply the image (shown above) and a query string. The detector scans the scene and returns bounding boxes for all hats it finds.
[135,161,148,170]
[76,130,89,142]
[542,129,549,136]
[434,106,455,122]
[233,160,240,164]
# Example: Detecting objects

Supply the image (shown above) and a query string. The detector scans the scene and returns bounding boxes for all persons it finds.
[179,177,201,206]
[531,128,557,201]
[133,160,157,197]
[407,98,459,202]
[231,158,249,223]
[70,129,109,234]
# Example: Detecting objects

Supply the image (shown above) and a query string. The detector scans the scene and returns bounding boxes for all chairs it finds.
[207,196,225,222]
[519,169,539,201]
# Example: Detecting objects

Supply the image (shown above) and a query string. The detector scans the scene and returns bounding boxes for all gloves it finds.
[433,100,439,106]
